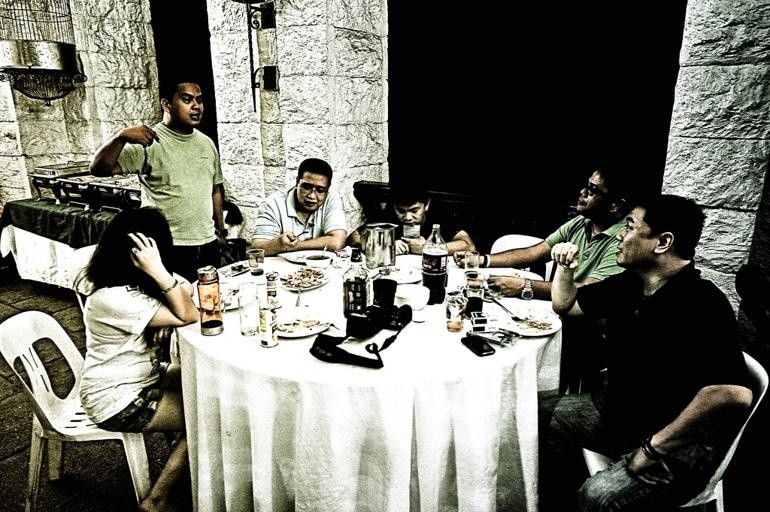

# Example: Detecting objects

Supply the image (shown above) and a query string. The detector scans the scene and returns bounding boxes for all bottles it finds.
[343,248,370,318]
[420,224,447,305]
[196,265,226,337]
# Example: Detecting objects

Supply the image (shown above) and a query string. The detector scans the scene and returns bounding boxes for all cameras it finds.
[347,279,412,339]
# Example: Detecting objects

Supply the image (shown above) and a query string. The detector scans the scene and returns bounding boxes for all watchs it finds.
[521,279,533,300]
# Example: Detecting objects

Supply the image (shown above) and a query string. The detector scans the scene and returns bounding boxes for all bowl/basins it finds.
[305,255,330,269]
[394,283,430,313]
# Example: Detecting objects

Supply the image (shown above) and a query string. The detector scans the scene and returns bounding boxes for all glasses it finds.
[579,183,615,200]
[296,181,329,194]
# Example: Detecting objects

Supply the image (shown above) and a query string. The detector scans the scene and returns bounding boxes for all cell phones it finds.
[461,336,495,357]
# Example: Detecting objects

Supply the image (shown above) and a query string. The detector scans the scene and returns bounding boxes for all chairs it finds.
[582,350,770,511]
[0,310,153,511]
[490,234,554,281]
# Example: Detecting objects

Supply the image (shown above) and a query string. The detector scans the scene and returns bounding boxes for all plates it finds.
[494,307,564,336]
[367,265,423,284]
[191,286,258,310]
[285,250,336,264]
[283,270,331,293]
[275,319,332,338]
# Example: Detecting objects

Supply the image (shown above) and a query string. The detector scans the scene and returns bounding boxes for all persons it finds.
[250,158,348,257]
[454,167,635,395]
[78,207,200,512]
[89,76,227,283]
[353,175,477,256]
[538,195,754,512]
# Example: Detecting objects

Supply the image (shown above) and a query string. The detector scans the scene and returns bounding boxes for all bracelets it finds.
[161,279,178,294]
[215,229,227,237]
[481,253,490,268]
[642,441,662,461]
[646,437,668,458]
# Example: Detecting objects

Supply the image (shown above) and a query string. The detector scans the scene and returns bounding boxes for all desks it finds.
[0,199,125,295]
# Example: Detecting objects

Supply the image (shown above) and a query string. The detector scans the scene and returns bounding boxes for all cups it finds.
[446,251,485,332]
[244,248,265,277]
[372,279,397,307]
[236,282,259,338]
[361,222,399,270]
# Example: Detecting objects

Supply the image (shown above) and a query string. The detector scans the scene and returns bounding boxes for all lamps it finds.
[232,0,279,114]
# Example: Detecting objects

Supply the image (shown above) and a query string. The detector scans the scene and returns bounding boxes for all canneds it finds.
[258,304,277,348]
[265,270,280,309]
